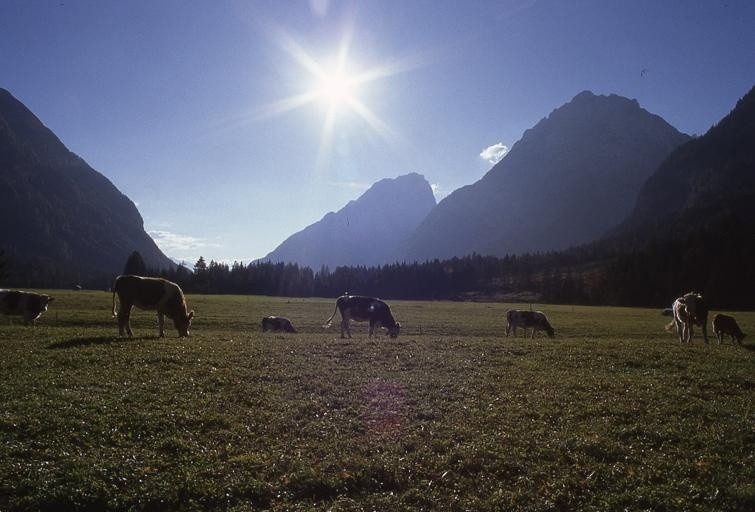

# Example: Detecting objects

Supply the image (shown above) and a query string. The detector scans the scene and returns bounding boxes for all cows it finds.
[322,295,400,339]
[262,316,298,333]
[0,290,55,328]
[664,291,710,346]
[505,310,555,340]
[711,313,748,345]
[111,275,194,338]
